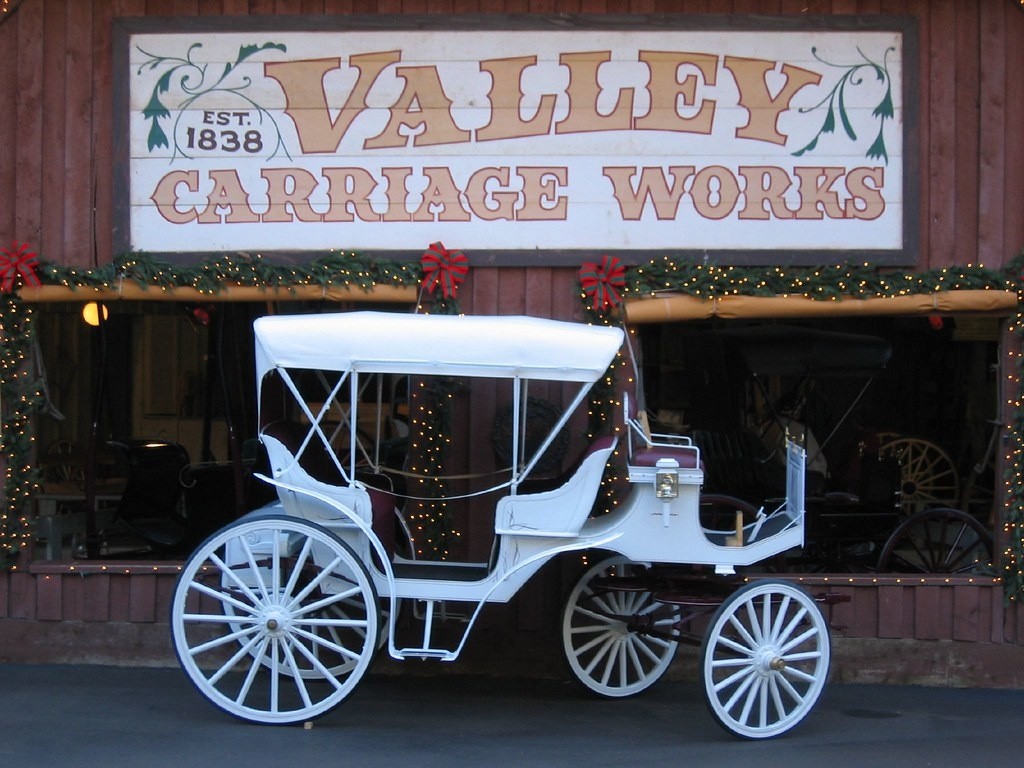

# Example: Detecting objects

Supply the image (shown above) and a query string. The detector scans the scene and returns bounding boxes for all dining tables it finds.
[37,478,128,560]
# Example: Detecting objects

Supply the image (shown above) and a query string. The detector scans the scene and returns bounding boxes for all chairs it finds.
[494,433,619,538]
[42,439,88,489]
[686,421,824,545]
[623,392,707,486]
[104,437,194,555]
[261,426,401,580]
